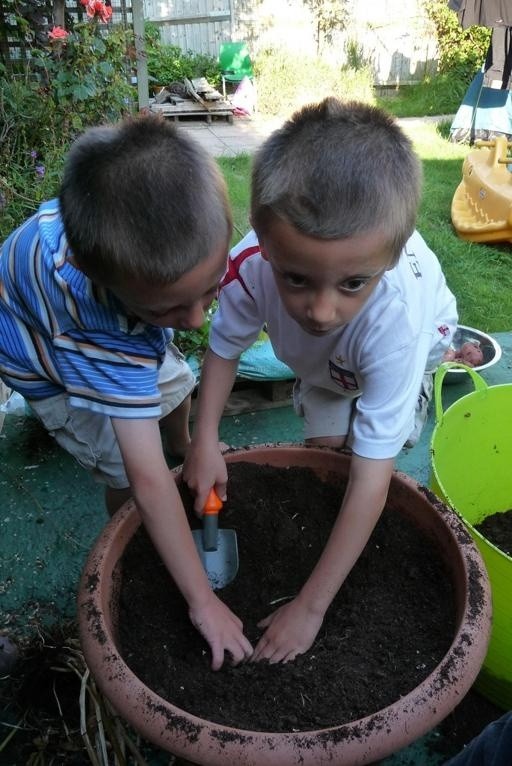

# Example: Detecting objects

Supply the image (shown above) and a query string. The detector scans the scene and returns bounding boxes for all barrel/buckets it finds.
[431,362,511,708]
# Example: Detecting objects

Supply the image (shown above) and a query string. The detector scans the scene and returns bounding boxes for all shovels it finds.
[191,485,238,591]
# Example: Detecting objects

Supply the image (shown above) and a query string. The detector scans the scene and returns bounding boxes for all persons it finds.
[0,103,253,673]
[178,97,461,671]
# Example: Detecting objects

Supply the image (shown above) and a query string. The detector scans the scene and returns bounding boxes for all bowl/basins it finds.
[436,324,502,385]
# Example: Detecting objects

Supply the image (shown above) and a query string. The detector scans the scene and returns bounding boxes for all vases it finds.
[74,441,492,766]
